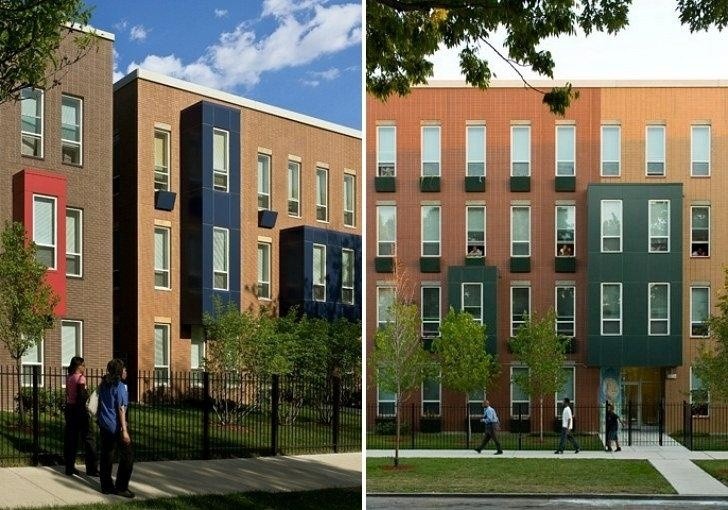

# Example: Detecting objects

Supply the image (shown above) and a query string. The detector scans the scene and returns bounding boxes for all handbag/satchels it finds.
[86,390,99,415]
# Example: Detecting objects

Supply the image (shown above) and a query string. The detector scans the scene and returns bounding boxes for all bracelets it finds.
[122,429,127,433]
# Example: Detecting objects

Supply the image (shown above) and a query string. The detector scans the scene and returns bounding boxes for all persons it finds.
[96,358,135,498]
[605,404,624,452]
[559,244,573,256]
[692,246,706,256]
[63,356,101,477]
[473,400,502,455]
[466,245,483,257]
[553,397,580,454]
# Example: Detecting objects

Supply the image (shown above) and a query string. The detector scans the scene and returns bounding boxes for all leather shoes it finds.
[67,469,101,476]
[555,450,563,454]
[494,451,503,455]
[474,448,480,452]
[575,447,581,453]
[608,446,621,451]
[104,487,135,497]
